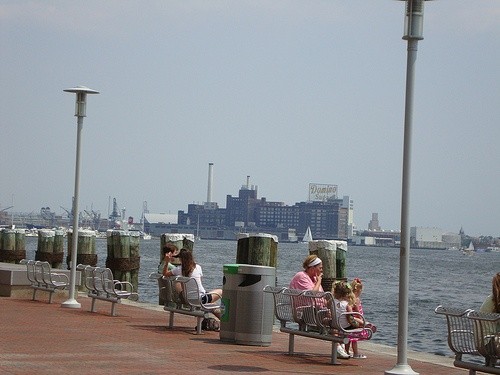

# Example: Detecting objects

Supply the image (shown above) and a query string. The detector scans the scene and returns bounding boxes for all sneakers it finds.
[336,344,352,359]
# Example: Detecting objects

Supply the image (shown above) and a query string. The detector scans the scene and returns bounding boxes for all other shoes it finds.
[353,353,367,358]
[364,322,376,333]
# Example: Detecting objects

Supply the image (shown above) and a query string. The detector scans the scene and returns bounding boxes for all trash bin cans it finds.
[220,264,275,345]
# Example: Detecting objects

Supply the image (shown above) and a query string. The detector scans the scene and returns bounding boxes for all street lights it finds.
[61,88,99,307]
[385,0,435,374]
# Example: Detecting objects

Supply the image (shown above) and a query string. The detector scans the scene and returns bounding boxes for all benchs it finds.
[434,304,500,375]
[263,285,372,364]
[147,272,222,334]
[20,259,70,304]
[75,264,139,316]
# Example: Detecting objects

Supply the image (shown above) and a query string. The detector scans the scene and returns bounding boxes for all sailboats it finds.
[300,226,313,243]
[463,241,475,252]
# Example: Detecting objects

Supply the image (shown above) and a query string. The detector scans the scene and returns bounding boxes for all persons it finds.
[290,255,350,359]
[345,278,367,359]
[158,243,178,281]
[474,272,500,365]
[325,281,376,333]
[163,249,222,332]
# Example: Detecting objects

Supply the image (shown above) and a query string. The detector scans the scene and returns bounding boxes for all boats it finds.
[0,224,152,240]
